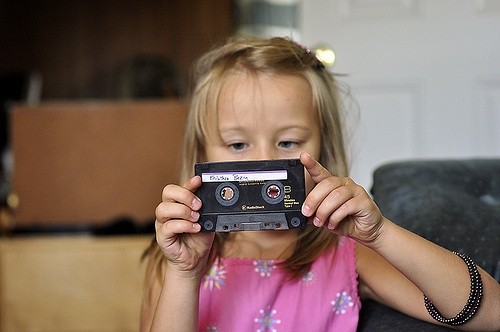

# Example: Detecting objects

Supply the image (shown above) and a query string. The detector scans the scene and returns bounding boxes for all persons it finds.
[139,33,500,332]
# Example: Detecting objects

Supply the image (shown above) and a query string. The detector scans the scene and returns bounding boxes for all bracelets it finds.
[424,251,485,327]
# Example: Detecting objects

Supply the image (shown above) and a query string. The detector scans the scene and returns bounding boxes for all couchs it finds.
[356,158,500,332]
